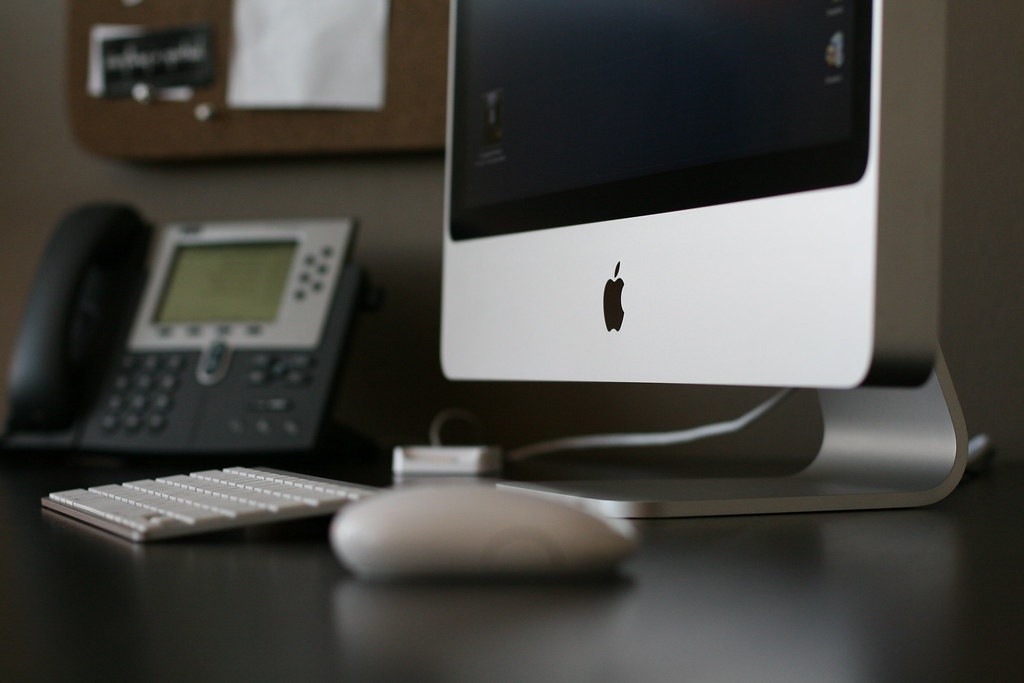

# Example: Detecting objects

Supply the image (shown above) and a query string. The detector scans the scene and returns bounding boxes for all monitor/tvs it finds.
[443,0,969,519]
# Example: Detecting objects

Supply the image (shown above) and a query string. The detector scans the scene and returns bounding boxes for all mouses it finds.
[329,478,638,581]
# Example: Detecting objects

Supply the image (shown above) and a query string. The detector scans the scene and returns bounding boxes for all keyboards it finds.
[41,465,383,541]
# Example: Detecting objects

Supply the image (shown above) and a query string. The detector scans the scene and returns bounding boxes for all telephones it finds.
[3,196,363,462]
